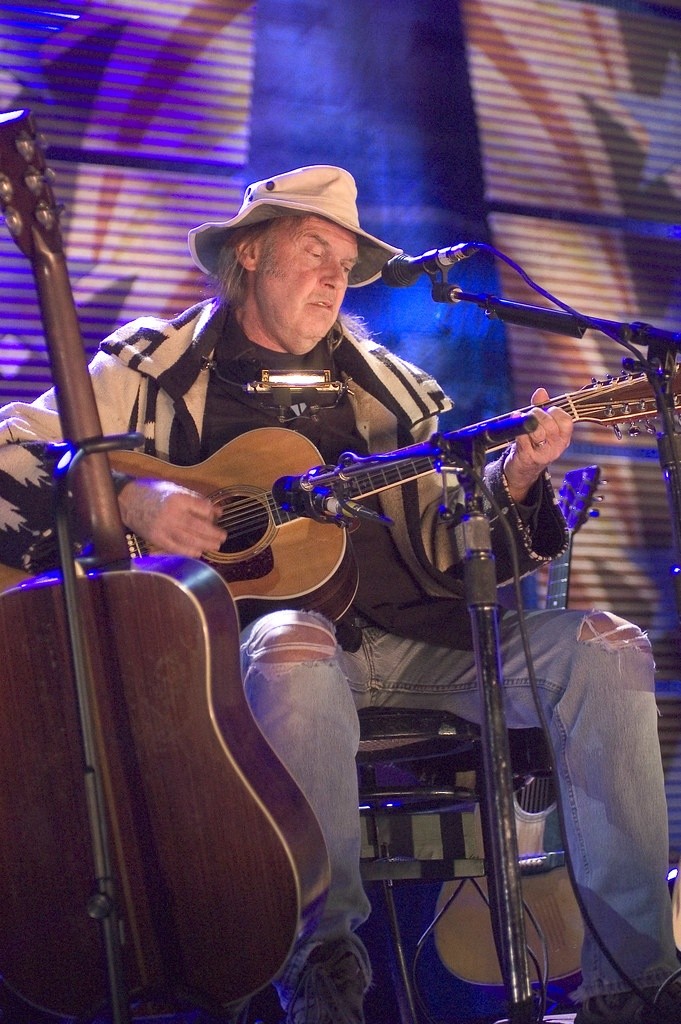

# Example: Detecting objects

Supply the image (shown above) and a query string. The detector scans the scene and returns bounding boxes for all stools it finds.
[354,707,554,1024]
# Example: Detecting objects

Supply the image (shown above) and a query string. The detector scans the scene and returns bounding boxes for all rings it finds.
[535,439,547,447]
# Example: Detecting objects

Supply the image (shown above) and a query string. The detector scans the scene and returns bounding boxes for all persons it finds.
[1,163,681,1023]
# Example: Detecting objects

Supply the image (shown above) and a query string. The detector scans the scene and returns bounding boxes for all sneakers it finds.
[573,984,681,1024]
[290,939,365,1024]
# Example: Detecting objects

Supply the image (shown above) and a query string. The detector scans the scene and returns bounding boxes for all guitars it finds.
[76,357,680,638]
[1,103,338,1024]
[430,463,611,989]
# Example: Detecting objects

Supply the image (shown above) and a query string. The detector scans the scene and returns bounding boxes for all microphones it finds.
[382,242,479,288]
[273,476,394,527]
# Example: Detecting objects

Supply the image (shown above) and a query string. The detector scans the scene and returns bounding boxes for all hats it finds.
[188,164,403,289]
